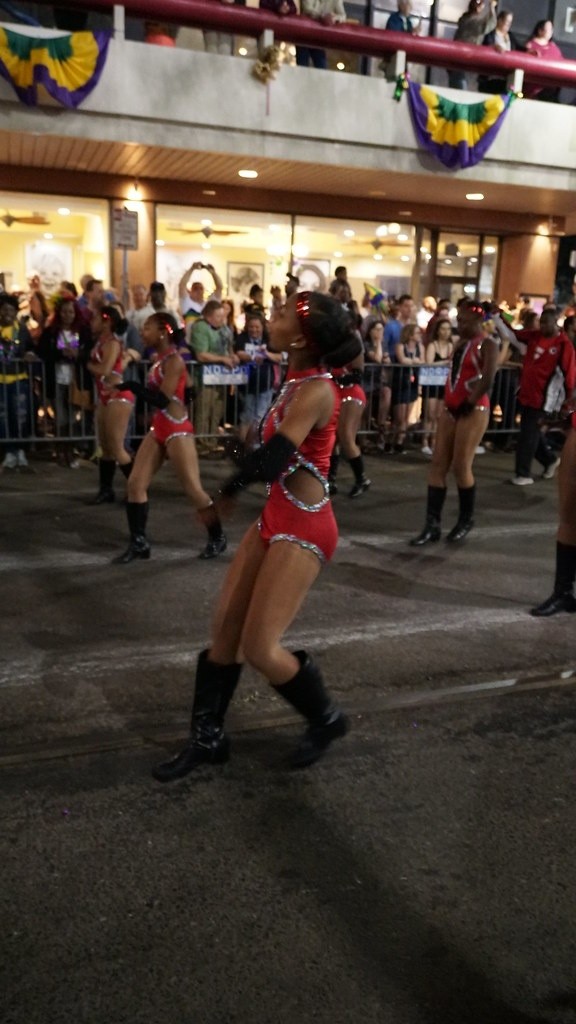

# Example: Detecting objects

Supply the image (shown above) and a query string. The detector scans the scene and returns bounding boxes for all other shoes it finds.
[395,443,408,455]
[384,444,394,455]
[422,446,432,455]
[16,449,28,466]
[3,452,18,468]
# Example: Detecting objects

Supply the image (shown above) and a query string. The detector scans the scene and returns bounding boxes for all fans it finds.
[0,211,50,228]
[170,219,246,238]
[343,237,412,253]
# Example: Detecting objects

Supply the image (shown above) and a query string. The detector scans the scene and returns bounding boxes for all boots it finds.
[447,482,475,540]
[85,459,117,506]
[119,458,135,479]
[529,541,576,616]
[113,500,151,564]
[197,498,226,559]
[410,484,447,545]
[327,455,339,495]
[146,649,243,782]
[269,651,351,767]
[347,455,370,498]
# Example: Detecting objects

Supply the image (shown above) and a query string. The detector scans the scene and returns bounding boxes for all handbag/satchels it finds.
[69,381,91,407]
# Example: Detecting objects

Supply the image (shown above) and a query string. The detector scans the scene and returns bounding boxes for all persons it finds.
[90,306,136,498]
[328,354,371,496]
[409,300,500,543]
[526,20,566,103]
[478,10,526,94]
[481,301,576,485]
[0,264,576,468]
[258,0,347,69]
[531,425,576,616]
[111,312,227,562]
[384,0,424,80]
[446,1,498,91]
[155,290,360,781]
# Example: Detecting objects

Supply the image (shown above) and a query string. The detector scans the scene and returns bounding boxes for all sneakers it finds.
[541,459,560,480]
[508,476,534,485]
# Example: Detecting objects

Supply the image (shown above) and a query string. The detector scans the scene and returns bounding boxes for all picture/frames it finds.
[291,257,331,293]
[225,261,265,300]
